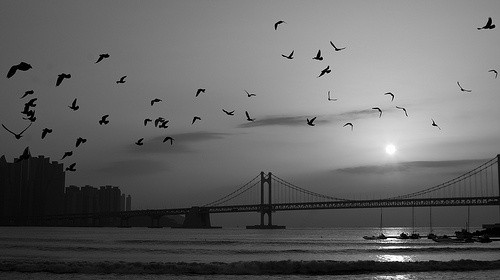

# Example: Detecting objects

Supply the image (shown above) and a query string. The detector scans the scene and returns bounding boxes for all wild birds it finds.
[281,50,295,59]
[56,73,72,87]
[456,81,472,92]
[245,110,256,122]
[133,116,176,147]
[343,122,354,130]
[191,115,202,125]
[195,88,207,98]
[116,75,127,85]
[244,89,257,98]
[306,116,317,126]
[41,127,53,140]
[396,106,409,117]
[488,69,498,78]
[384,92,395,101]
[476,16,496,30]
[274,20,287,31]
[312,49,324,61]
[328,90,338,102]
[60,136,87,172]
[150,97,162,107]
[329,40,346,51]
[67,97,80,112]
[1,90,38,141]
[5,61,33,79]
[95,53,111,65]
[98,113,110,126]
[222,109,235,116]
[431,119,440,130]
[317,65,331,78]
[372,107,383,119]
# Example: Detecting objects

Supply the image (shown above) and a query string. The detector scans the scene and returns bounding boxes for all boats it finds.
[427,233,437,239]
[375,233,387,239]
[409,234,421,239]
[432,223,500,243]
[363,236,377,240]
[397,232,410,239]
